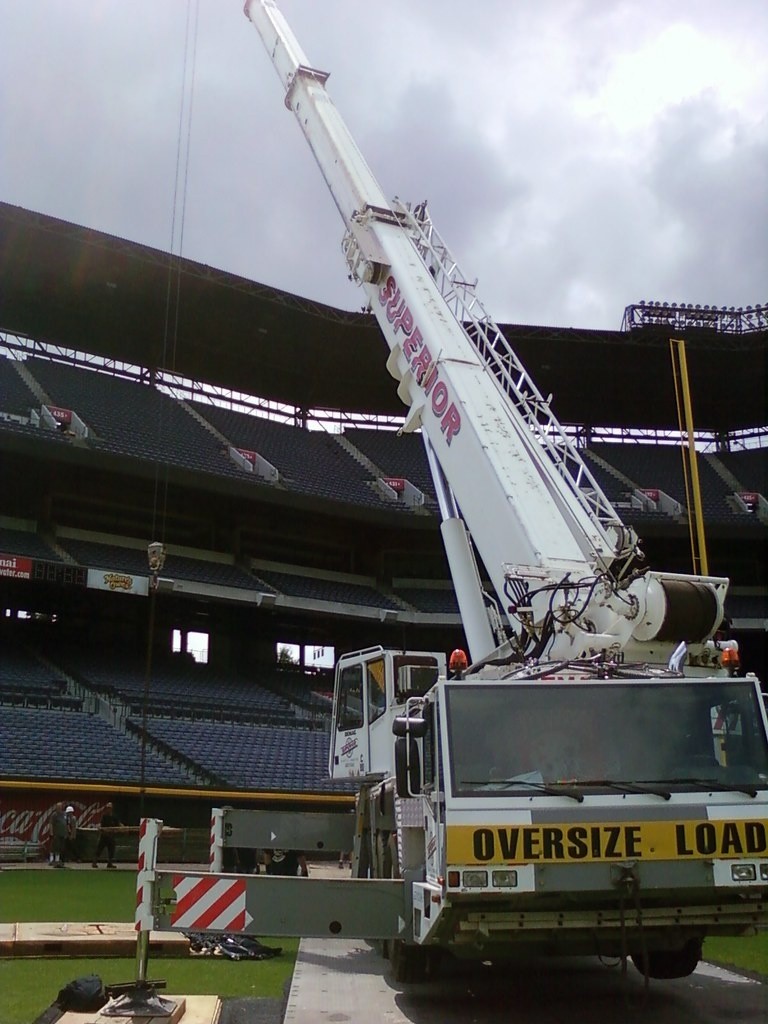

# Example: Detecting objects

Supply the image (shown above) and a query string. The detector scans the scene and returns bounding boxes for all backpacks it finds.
[58,973,106,1013]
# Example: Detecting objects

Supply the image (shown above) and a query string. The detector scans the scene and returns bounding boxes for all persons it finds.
[47,802,81,868]
[91,802,124,868]
[220,806,357,877]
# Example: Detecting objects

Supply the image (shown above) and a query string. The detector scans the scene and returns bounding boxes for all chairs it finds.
[0,651,361,794]
[0,355,768,522]
[0,528,505,615]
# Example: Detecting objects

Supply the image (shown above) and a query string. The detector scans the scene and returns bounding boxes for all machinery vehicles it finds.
[99,0,768,1016]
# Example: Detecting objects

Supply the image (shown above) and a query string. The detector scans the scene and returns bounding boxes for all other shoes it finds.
[49,860,55,866]
[56,860,64,865]
[107,863,117,868]
[92,863,98,868]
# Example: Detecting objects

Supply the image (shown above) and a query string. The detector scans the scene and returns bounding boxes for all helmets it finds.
[65,806,74,813]
